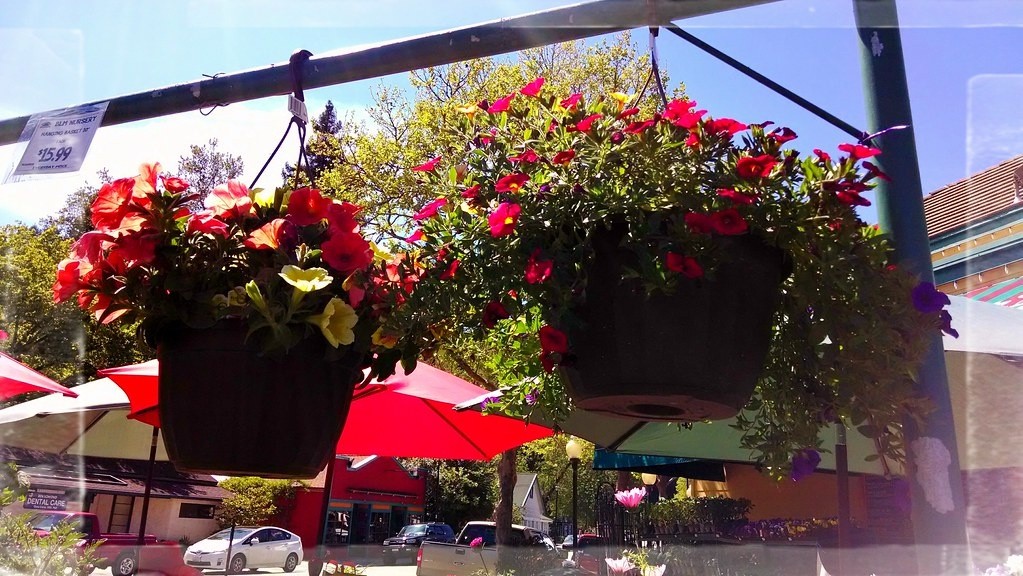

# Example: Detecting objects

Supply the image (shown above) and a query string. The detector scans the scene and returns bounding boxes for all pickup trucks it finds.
[21,510,183,576]
[416,521,557,576]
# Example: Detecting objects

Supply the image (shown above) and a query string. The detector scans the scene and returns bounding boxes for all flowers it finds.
[51,77,961,491]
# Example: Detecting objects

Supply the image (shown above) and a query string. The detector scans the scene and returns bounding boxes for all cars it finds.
[183,526,304,575]
[562,534,610,549]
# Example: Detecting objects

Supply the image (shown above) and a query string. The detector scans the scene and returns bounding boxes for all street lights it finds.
[641,472,656,504]
[566,439,581,548]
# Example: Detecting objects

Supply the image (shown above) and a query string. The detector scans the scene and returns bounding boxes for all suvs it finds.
[381,521,457,565]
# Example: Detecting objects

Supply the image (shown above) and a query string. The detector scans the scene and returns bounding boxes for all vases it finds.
[544,213,797,426]
[155,319,365,483]
